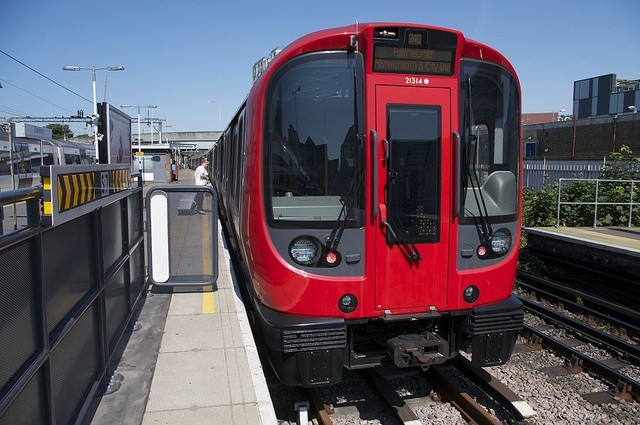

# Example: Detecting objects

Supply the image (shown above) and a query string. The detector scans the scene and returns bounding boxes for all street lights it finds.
[163,125,172,144]
[62,65,124,163]
[120,105,157,175]
[132,117,166,145]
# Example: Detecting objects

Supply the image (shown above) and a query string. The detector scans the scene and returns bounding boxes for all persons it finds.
[194,158,214,215]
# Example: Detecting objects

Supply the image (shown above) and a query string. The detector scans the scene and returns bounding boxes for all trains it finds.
[188,23,527,402]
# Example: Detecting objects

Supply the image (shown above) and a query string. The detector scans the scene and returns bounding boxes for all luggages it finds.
[176,192,198,216]
